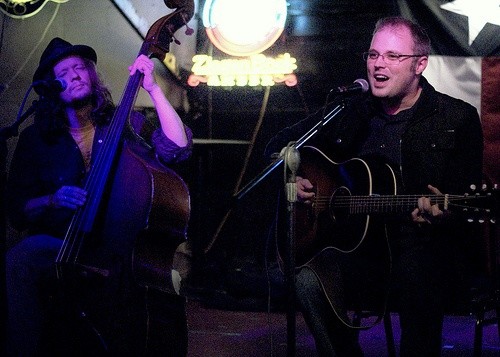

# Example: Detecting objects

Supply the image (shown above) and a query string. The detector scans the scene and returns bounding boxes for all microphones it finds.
[330,79,369,93]
[33,78,67,92]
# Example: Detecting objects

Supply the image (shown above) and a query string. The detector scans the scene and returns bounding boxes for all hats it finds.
[31,37,97,98]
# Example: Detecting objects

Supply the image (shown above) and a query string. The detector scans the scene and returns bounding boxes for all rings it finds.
[64,196,66,200]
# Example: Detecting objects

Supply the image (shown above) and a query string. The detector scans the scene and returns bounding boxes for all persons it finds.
[262,16,485,357]
[5,35,197,356]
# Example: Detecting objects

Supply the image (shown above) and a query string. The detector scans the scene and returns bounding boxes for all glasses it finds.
[363,51,423,65]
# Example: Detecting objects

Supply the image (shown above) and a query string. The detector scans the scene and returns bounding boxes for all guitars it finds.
[273,145,500,268]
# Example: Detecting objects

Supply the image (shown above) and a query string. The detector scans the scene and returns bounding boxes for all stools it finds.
[348,303,483,357]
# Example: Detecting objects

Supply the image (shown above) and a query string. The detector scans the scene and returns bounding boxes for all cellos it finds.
[34,0,193,357]
